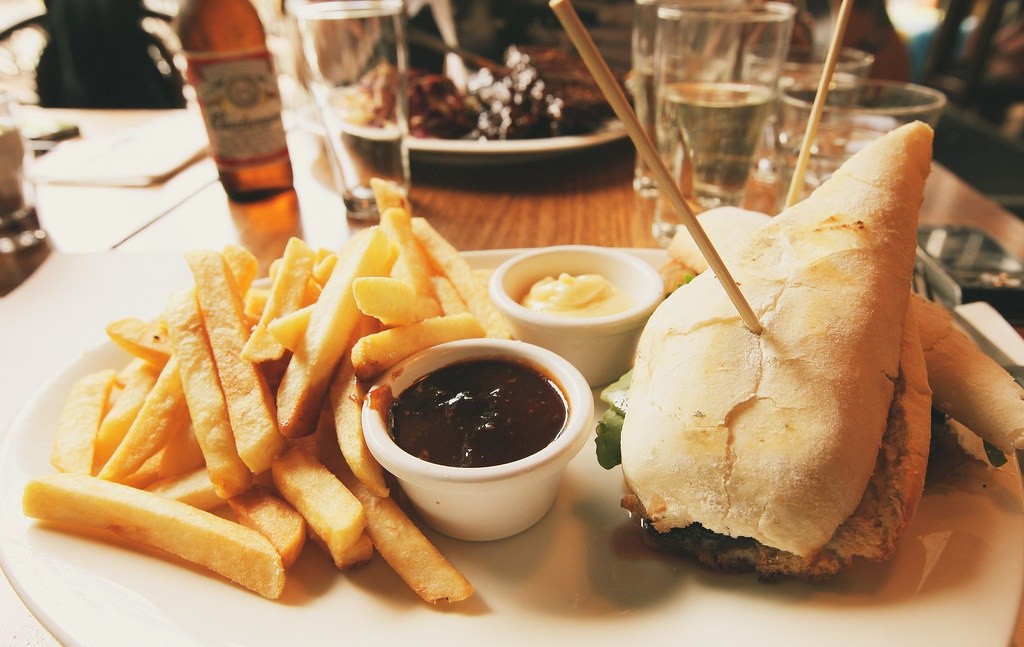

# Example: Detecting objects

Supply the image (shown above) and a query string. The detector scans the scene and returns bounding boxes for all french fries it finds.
[16,178,513,602]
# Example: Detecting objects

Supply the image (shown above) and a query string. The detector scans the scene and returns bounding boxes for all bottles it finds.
[171,0,294,200]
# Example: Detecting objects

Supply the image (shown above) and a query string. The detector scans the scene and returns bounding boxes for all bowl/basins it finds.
[364,244,658,540]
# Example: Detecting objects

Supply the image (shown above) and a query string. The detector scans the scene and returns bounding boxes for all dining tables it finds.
[0,0,1022,647]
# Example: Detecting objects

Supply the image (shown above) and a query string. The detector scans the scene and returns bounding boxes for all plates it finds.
[0,247,1024,646]
[343,99,628,164]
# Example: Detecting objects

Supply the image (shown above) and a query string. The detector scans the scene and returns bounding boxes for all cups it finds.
[295,0,413,217]
[2,90,44,250]
[622,0,946,253]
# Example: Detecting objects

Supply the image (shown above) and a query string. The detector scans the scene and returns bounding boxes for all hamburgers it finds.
[597,120,1024,587]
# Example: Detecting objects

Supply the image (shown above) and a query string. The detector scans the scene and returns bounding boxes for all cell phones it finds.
[914,221,1023,317]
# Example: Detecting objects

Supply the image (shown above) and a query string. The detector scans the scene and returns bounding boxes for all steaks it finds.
[359,46,621,139]
